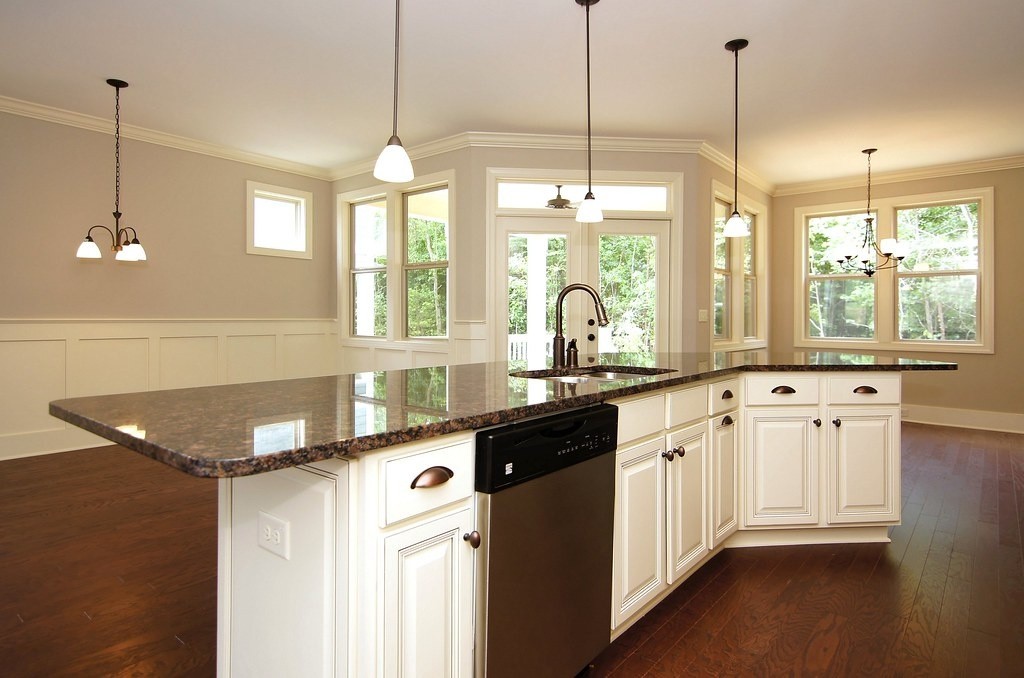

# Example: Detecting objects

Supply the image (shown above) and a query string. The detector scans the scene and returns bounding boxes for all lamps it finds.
[545,186,576,209]
[836,149,906,277]
[77,79,147,262]
[722,38,751,237]
[575,0,604,223]
[373,0,414,184]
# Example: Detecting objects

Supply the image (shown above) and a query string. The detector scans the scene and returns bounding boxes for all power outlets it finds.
[257,511,290,560]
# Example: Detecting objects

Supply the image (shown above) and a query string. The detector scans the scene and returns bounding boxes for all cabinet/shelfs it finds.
[611,382,707,630]
[743,376,902,530]
[708,378,738,550]
[216,435,480,677]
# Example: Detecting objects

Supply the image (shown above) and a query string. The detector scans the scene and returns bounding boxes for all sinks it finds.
[581,371,650,379]
[532,376,587,384]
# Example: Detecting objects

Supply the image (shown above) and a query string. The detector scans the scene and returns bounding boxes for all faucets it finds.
[553,283,609,367]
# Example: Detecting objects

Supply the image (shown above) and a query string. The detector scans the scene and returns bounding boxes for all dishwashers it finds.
[474,403,619,678]
